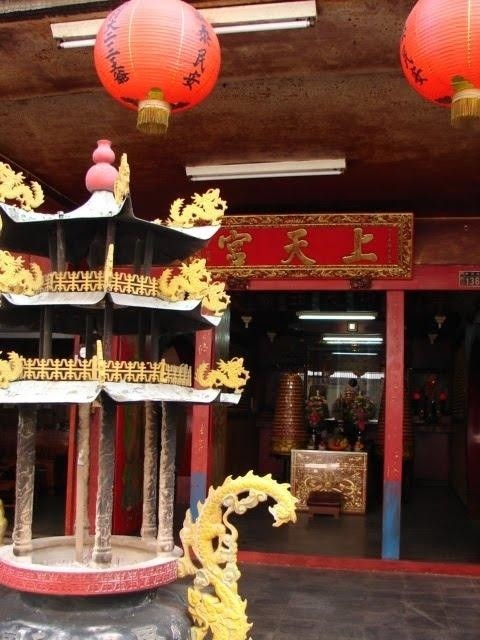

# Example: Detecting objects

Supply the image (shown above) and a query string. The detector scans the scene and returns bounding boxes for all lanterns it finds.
[91,0,222,135]
[398,0,480,131]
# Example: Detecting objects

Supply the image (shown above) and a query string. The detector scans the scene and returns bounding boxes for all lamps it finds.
[184,153,352,187]
[295,307,378,325]
[317,330,384,346]
[43,2,317,51]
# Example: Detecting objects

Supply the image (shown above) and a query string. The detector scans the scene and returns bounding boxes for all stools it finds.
[306,492,344,523]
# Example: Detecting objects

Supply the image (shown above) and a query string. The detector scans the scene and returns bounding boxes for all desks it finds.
[289,447,369,517]
